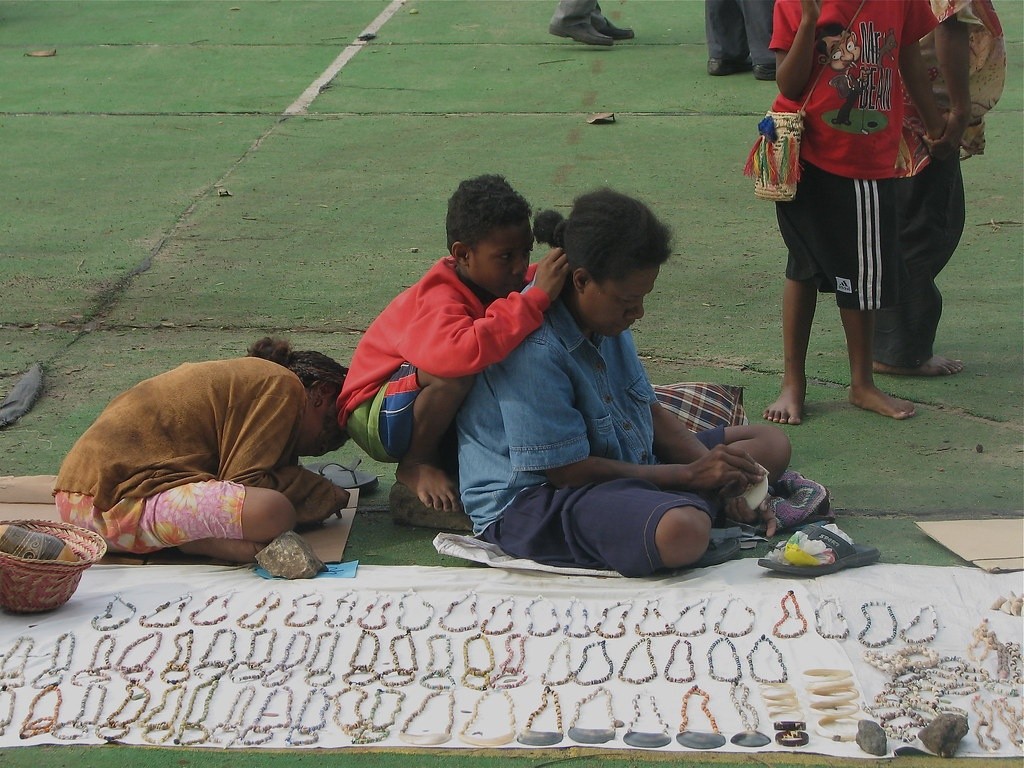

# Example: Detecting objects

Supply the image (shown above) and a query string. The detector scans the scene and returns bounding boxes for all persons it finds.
[338,175,570,514]
[549,0,634,45]
[761,0,1006,424]
[51,337,349,564]
[456,190,791,576]
[704,0,776,81]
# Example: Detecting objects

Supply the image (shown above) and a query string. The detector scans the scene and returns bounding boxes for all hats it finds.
[0,518,107,613]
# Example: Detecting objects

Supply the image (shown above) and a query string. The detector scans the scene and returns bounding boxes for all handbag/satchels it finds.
[745,109,804,200]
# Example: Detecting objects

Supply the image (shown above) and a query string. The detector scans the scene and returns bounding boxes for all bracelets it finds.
[865,645,989,742]
[758,668,861,746]
[18,716,390,747]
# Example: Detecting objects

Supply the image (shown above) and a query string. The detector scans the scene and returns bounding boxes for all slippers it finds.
[695,535,742,572]
[304,455,378,490]
[758,523,881,576]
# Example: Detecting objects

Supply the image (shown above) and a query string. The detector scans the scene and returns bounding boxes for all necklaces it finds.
[730,682,771,747]
[399,589,726,748]
[747,634,787,683]
[815,598,849,640]
[707,637,742,682]
[969,618,1024,752]
[0,590,435,733]
[858,601,897,648]
[898,605,938,644]
[714,593,756,638]
[771,590,807,638]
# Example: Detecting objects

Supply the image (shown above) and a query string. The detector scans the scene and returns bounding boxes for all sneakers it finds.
[708,54,754,75]
[598,17,634,38]
[549,22,613,46]
[755,64,777,80]
